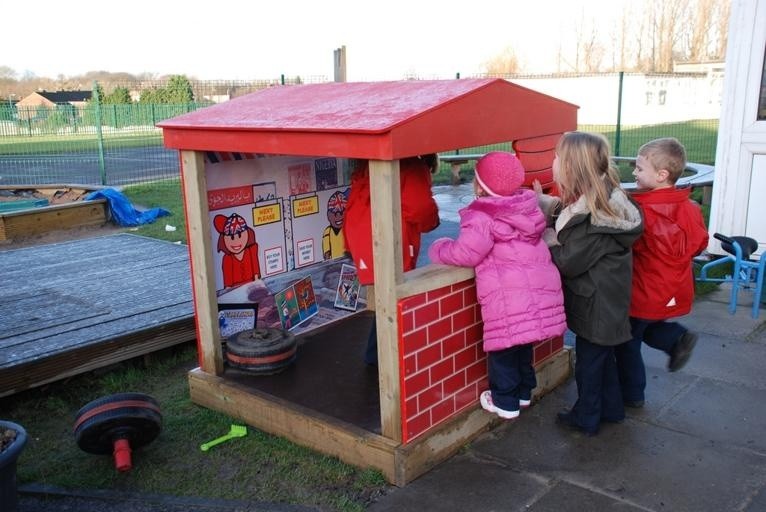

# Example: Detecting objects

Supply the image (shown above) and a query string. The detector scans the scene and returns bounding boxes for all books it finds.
[274,273,319,332]
[333,263,361,311]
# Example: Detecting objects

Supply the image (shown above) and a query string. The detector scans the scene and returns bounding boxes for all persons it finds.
[529,130,645,434]
[319,267,341,310]
[425,150,570,422]
[618,137,711,409]
[339,150,443,369]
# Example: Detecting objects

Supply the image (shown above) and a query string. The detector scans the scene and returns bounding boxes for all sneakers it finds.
[557,400,645,433]
[480,390,531,420]
[667,331,698,373]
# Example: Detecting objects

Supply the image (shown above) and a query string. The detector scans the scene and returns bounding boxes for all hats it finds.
[474,150,525,196]
